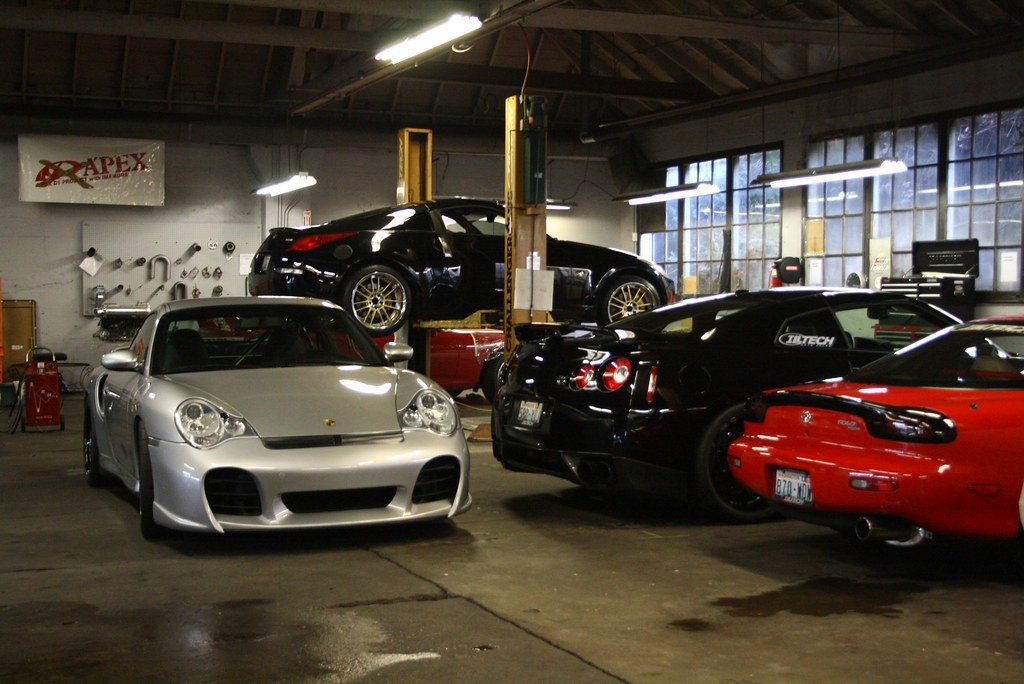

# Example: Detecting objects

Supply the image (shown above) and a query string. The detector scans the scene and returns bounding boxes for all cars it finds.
[491,284,1013,526]
[728,316,1023,579]
[82,296,473,540]
[248,195,676,336]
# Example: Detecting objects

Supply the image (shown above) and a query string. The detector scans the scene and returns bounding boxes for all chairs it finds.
[973,355,1020,381]
[163,330,218,373]
[264,326,321,369]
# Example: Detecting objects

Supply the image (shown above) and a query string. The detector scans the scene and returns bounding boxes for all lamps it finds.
[544,159,576,210]
[250,143,319,197]
[751,0,908,187]
[375,11,483,65]
[611,101,720,205]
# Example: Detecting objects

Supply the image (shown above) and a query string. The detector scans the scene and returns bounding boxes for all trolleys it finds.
[21,345,65,432]
